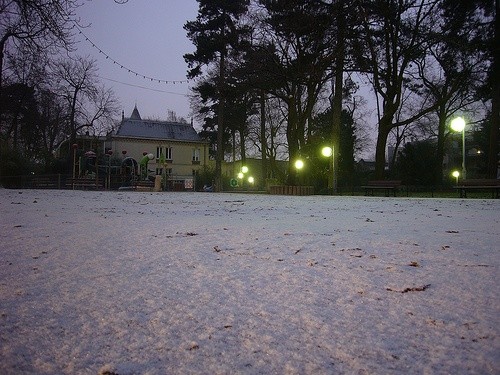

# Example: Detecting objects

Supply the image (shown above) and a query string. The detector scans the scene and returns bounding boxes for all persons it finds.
[155,150,165,175]
[140,152,149,181]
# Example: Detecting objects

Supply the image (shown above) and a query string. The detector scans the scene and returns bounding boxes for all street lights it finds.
[320,145,335,195]
[108,150,113,191]
[121,150,127,158]
[449,115,466,198]
[294,159,305,185]
[143,151,149,177]
[72,143,78,190]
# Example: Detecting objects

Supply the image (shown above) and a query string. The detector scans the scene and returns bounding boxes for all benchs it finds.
[450,178,500,199]
[430,183,464,198]
[361,179,401,197]
[64,178,105,193]
[30,174,62,189]
